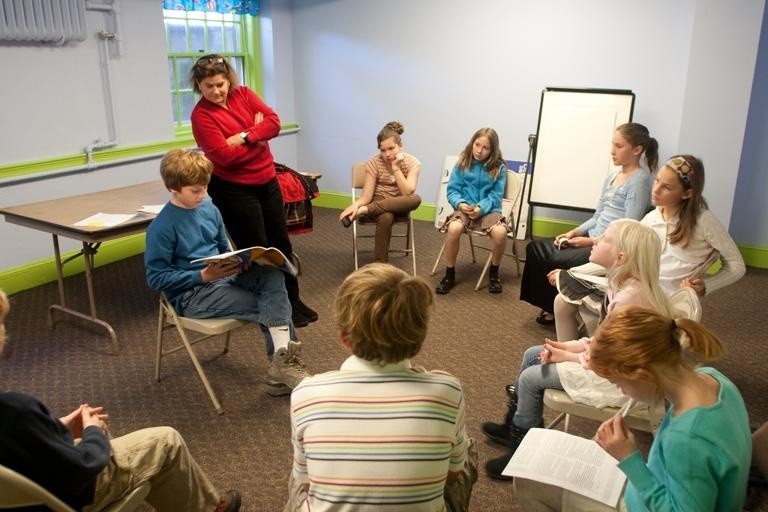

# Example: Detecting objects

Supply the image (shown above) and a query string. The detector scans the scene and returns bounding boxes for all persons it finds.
[512,304,754,512]
[518,122,659,326]
[479,217,676,481]
[143,147,314,398]
[337,122,423,263]
[283,261,480,512]
[546,155,748,342]
[189,53,318,328]
[434,126,509,295]
[0,289,242,511]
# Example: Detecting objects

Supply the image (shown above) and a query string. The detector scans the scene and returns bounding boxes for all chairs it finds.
[429,167,523,292]
[536,288,701,450]
[346,160,416,280]
[0,464,158,512]
[147,225,253,416]
[574,249,721,341]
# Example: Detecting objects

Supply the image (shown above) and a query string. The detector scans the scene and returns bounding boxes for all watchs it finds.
[240,130,249,144]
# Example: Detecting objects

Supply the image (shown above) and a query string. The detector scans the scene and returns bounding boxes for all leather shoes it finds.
[489,275,502,292]
[506,385,517,398]
[536,315,555,325]
[215,490,241,512]
[436,276,455,293]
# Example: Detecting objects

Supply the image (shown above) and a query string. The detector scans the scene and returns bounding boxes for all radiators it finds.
[0,0,90,47]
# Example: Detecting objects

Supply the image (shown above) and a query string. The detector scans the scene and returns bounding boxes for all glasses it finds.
[198,56,223,66]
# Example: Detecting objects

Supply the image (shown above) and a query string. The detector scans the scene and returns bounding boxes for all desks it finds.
[0,169,326,353]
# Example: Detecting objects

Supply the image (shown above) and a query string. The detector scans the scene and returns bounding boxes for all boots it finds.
[481,419,544,481]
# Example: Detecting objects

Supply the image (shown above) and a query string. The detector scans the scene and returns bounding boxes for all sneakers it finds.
[266,341,311,396]
[343,216,350,227]
[292,301,317,326]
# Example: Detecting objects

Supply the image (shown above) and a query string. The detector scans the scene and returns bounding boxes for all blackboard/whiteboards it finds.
[526,87,634,213]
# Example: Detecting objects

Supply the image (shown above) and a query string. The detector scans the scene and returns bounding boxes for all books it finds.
[190,245,298,279]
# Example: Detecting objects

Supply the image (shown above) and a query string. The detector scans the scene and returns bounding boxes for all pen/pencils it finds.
[623,397,634,418]
